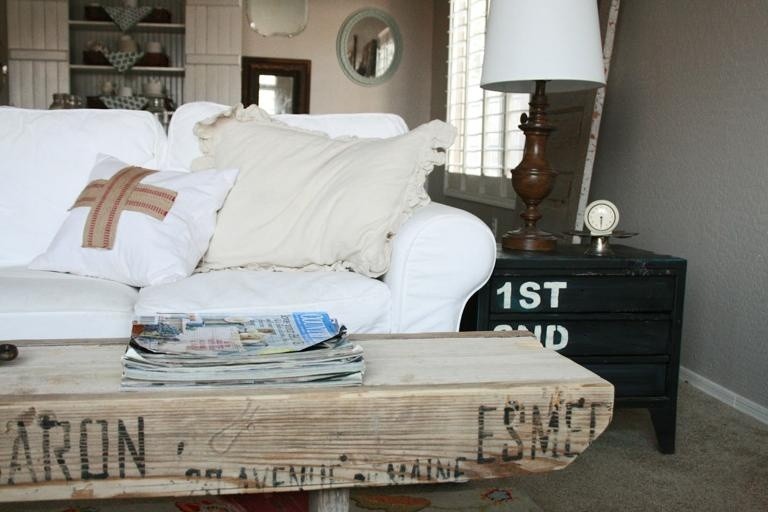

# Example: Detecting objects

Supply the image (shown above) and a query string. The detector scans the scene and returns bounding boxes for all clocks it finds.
[584,200,622,236]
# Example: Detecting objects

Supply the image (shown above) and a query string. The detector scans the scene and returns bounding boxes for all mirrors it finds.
[337,7,404,87]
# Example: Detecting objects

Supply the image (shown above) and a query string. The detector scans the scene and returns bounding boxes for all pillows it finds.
[190,102,458,279]
[25,150,242,286]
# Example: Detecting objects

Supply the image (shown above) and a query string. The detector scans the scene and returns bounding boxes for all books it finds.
[120,312,365,389]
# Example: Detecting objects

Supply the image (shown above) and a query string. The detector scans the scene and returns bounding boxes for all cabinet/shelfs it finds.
[65,0,186,114]
[466,245,688,456]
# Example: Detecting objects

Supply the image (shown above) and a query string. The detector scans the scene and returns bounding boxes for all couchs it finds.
[0,101,496,341]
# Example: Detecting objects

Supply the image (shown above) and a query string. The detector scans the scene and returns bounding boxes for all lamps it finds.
[481,0,608,252]
